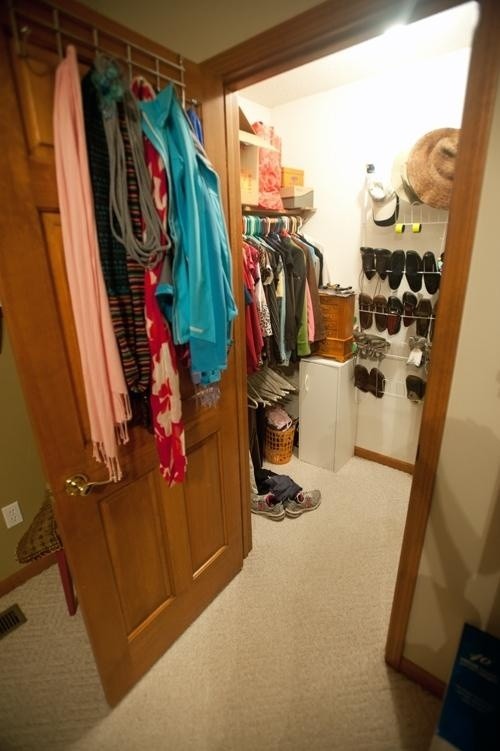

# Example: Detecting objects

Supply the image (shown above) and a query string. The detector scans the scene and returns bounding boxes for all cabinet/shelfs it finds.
[297,355,357,473]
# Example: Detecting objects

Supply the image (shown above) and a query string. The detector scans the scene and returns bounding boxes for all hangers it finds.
[247,356,299,409]
[240,213,317,255]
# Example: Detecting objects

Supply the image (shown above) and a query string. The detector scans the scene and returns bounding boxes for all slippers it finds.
[368,367,385,398]
[354,365,369,393]
[406,374,425,405]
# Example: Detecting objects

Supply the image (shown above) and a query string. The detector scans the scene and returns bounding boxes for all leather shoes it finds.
[360,246,440,294]
[359,294,373,330]
[416,298,432,338]
[403,291,418,327]
[387,296,403,335]
[373,295,388,332]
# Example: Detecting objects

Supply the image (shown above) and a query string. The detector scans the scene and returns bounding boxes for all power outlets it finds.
[0,500,23,531]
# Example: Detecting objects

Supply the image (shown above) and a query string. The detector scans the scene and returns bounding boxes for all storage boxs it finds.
[239,106,313,211]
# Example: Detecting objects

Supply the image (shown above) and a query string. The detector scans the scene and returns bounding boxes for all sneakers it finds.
[252,493,285,521]
[285,489,322,518]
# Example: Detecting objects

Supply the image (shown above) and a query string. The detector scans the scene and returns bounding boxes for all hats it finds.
[392,153,424,206]
[407,128,459,210]
[367,172,397,221]
